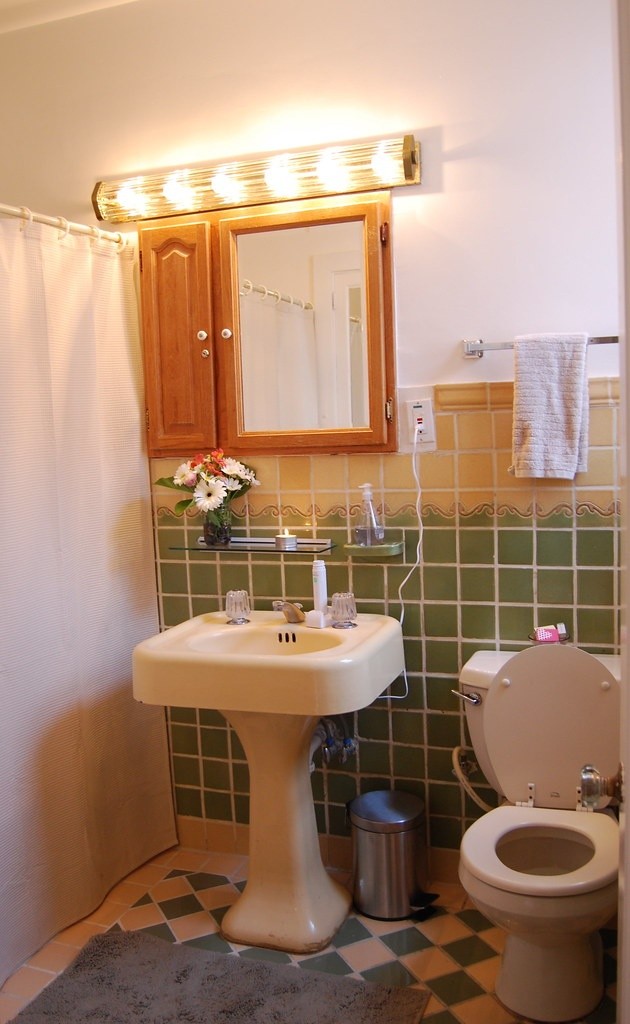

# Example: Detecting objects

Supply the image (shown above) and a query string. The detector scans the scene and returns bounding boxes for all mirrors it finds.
[235,217,371,430]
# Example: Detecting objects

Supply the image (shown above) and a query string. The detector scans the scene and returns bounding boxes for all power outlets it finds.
[407,398,435,444]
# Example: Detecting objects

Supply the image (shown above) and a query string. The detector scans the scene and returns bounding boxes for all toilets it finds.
[451,644,619,1022]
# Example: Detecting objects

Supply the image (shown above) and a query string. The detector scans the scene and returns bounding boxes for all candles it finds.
[275,528,297,549]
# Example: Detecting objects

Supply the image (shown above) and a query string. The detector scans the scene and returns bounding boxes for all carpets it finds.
[6,930,433,1024]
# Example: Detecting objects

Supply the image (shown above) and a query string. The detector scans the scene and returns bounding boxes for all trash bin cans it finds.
[349,789,427,920]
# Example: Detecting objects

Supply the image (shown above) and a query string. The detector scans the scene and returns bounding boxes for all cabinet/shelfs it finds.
[140,201,400,459]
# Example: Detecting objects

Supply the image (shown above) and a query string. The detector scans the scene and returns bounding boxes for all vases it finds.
[203,509,231,546]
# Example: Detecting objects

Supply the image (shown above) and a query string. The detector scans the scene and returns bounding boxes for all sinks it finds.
[134,609,405,714]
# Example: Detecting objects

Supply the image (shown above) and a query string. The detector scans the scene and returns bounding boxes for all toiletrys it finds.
[354,483,385,545]
[311,561,327,614]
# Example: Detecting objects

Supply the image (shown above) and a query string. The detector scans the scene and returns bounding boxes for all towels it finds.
[511,332,588,480]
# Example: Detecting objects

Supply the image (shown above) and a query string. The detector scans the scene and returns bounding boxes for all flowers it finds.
[153,448,262,526]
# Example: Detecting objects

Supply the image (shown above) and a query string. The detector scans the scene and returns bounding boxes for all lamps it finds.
[91,132,421,226]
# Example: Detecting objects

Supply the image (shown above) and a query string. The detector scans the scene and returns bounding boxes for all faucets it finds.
[273,600,305,624]
